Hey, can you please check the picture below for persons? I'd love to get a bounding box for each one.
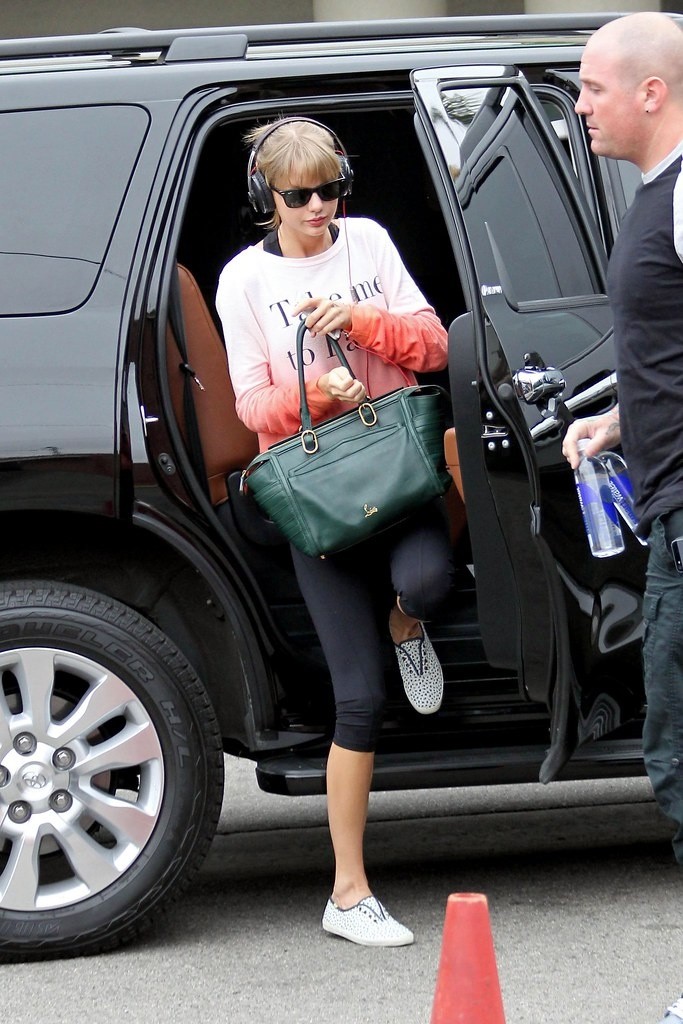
[562,14,683,859]
[205,114,472,945]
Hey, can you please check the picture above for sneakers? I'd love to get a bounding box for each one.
[391,619,444,714]
[322,897,413,947]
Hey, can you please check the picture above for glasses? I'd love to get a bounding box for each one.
[267,171,348,207]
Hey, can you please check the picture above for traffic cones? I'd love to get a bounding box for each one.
[429,891,508,1023]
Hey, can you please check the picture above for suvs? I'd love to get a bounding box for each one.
[0,8,682,964]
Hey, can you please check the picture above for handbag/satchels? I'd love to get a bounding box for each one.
[238,317,452,560]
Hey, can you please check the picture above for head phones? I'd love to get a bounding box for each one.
[247,116,355,214]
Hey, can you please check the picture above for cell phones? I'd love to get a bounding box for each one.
[293,294,342,341]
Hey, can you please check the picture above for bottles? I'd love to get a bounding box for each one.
[573,437,626,558]
[595,445,651,548]
